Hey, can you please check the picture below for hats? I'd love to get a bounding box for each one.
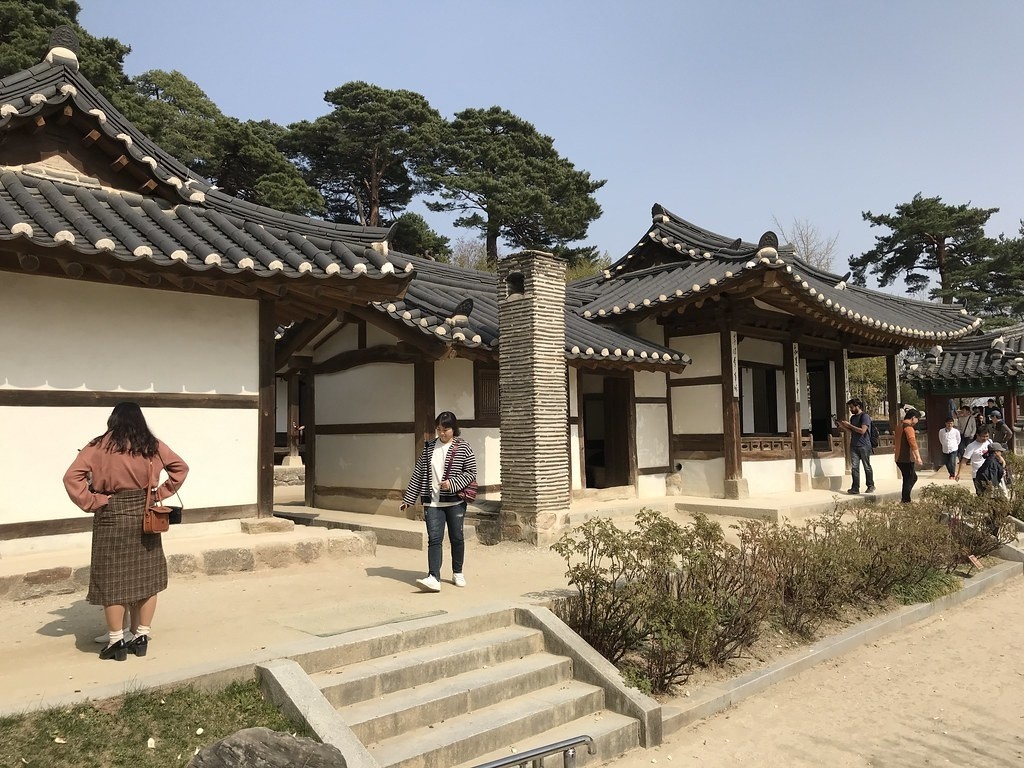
[988,443,1004,452]
[988,410,1001,417]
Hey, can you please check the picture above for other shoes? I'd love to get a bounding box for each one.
[865,486,875,493]
[848,488,859,494]
[966,460,972,465]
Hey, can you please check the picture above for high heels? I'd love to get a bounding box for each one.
[126,635,148,656]
[99,639,128,660]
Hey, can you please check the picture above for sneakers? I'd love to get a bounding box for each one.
[95,627,129,643]
[123,630,134,642]
[452,572,465,586]
[416,574,441,593]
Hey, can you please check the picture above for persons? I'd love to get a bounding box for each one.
[834,398,876,495]
[62,402,190,661]
[991,410,1013,448]
[399,411,477,592]
[895,409,922,503]
[938,417,962,479]
[977,443,1009,499]
[956,399,1003,465]
[963,424,994,485]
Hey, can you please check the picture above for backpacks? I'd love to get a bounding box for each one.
[851,413,879,447]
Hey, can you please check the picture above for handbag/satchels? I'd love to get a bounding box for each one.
[961,433,965,442]
[165,506,182,524]
[142,506,173,533]
[445,438,478,503]
